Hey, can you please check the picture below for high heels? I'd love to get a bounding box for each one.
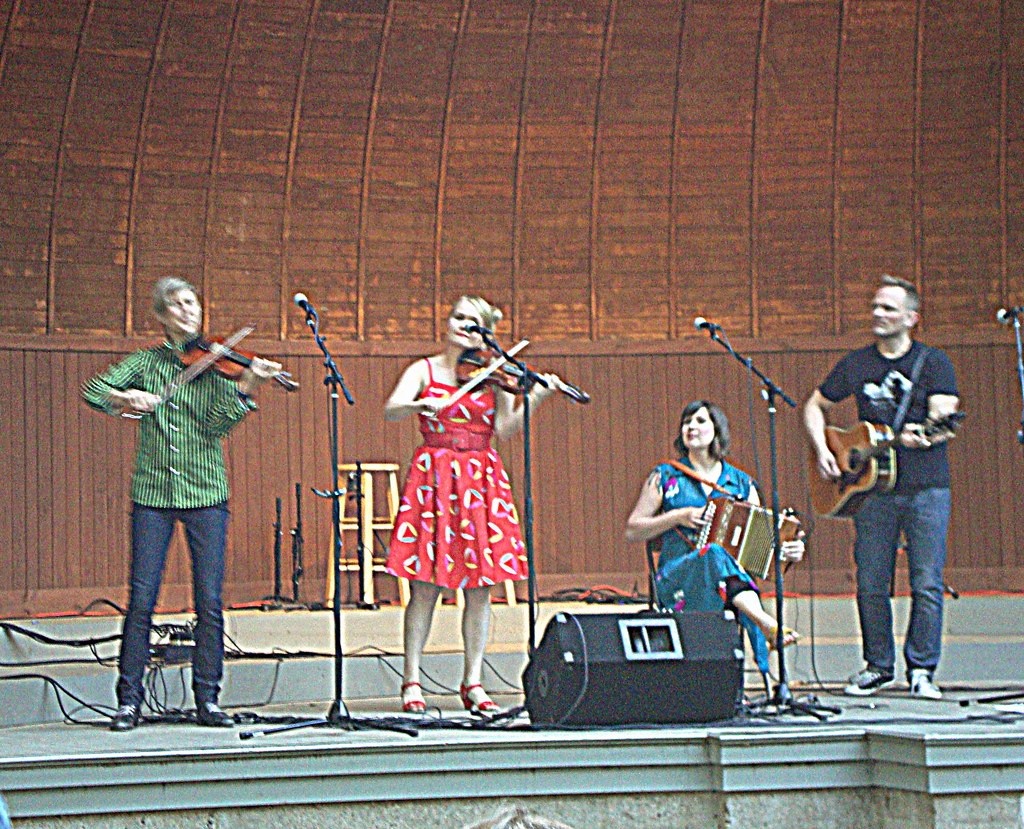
[400,680,427,714]
[459,681,501,712]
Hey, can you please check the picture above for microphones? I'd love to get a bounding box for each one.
[294,292,317,317]
[996,307,1023,324]
[465,321,493,335]
[693,317,721,332]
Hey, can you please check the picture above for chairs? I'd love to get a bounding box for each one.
[637,528,773,700]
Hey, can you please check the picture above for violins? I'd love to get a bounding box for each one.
[453,344,591,405]
[175,332,302,393]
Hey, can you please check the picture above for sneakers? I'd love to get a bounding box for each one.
[195,702,235,727]
[110,704,142,731]
[844,668,895,695]
[906,668,942,700]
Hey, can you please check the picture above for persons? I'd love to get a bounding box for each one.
[625,399,806,675]
[803,276,959,701]
[382,295,560,713]
[77,277,283,732]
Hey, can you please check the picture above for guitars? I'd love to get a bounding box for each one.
[805,407,973,520]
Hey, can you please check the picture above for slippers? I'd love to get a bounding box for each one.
[771,628,802,650]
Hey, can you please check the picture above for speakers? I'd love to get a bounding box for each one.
[522,610,745,729]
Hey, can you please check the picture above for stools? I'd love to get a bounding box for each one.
[325,460,410,606]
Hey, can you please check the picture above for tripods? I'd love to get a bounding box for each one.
[705,331,841,722]
[238,316,418,741]
